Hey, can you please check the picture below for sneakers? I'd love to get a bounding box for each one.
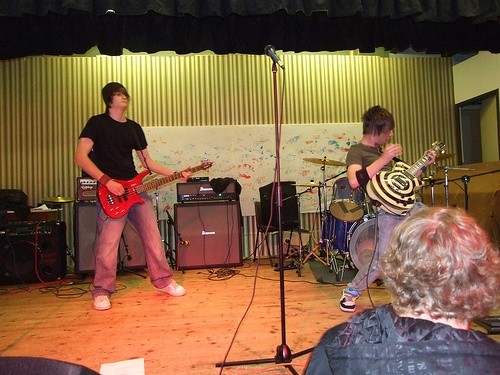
[153,278,186,297]
[339,288,360,312]
[92,295,112,311]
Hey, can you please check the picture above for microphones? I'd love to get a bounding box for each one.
[263,45,285,70]
[179,239,190,246]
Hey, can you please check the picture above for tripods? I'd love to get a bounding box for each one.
[214,62,315,375]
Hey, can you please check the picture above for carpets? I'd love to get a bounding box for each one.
[307,256,383,284]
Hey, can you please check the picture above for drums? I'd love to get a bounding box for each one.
[318,210,354,254]
[328,177,365,223]
[346,214,379,270]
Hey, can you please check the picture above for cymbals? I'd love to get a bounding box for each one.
[291,183,333,188]
[41,196,75,204]
[428,152,457,163]
[302,158,347,166]
[420,177,446,183]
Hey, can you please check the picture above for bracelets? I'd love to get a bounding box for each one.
[356,167,370,186]
[99,174,111,186]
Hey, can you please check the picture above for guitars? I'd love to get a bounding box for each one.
[366,141,446,217]
[97,159,213,219]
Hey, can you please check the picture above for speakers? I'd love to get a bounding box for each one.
[74,202,147,273]
[0,232,66,285]
[173,201,243,268]
[260,181,299,228]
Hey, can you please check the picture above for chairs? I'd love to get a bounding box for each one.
[252,201,278,268]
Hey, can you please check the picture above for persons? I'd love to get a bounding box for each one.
[340,104,437,311]
[75,82,190,310]
[304,206,500,375]
